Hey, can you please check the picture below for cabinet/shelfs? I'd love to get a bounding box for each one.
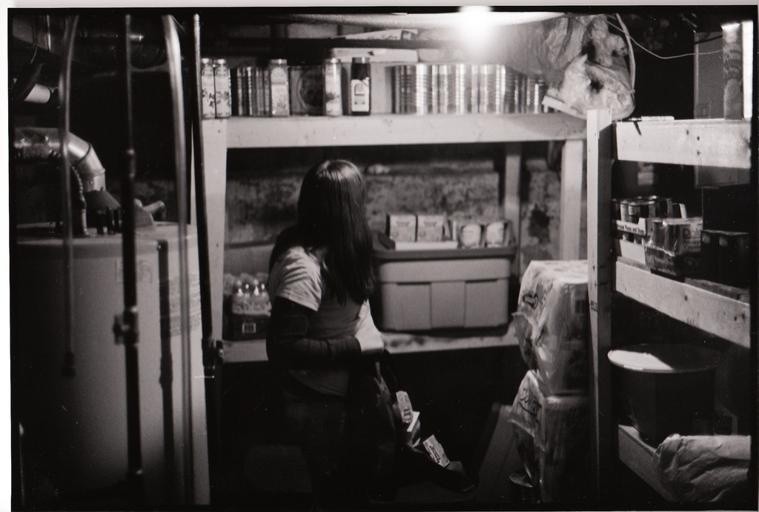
[197,109,584,512]
[587,111,759,511]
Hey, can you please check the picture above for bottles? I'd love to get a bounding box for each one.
[269,59,290,116]
[350,56,371,116]
[213,58,232,118]
[321,58,343,116]
[201,58,216,118]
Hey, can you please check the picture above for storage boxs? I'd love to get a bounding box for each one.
[373,228,511,332]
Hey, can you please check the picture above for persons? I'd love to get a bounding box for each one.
[265,158,385,399]
